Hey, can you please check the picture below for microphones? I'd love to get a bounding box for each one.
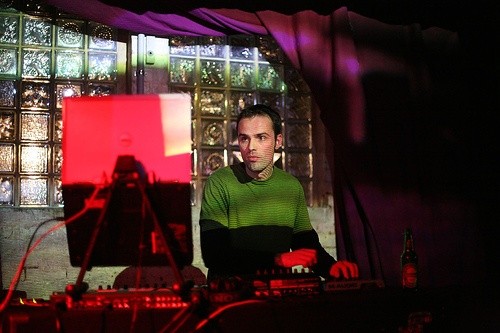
[401,230,419,288]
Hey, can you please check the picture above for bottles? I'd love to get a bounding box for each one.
[400,228,417,292]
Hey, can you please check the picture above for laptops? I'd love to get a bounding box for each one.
[63,93,195,268]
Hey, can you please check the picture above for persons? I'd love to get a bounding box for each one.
[200,105,359,279]
